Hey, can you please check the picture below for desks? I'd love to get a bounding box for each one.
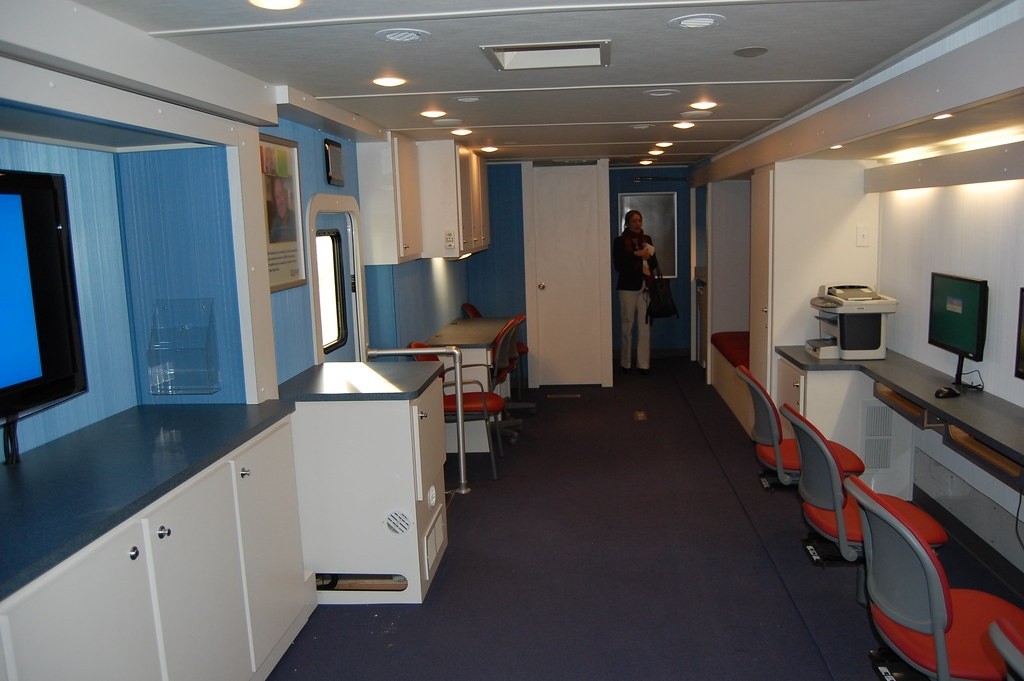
[425,315,514,393]
[775,346,1024,495]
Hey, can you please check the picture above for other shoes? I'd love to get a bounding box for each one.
[638,368,652,375]
[621,367,631,374]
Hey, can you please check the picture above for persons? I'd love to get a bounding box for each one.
[613,210,659,375]
[268,176,297,241]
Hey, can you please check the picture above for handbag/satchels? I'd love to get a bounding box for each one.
[642,253,679,324]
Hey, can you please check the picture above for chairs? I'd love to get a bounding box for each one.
[408,302,528,482]
[844,477,1024,681]
[779,405,948,606]
[735,362,865,485]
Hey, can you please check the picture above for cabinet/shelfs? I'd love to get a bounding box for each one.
[0,379,448,681]
[357,140,491,266]
[776,358,914,501]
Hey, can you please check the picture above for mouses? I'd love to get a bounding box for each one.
[934,386,962,398]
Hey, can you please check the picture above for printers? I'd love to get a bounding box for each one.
[805,283,899,360]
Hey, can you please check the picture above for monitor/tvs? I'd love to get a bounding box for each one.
[927,272,989,385]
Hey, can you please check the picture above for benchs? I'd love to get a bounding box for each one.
[710,332,755,440]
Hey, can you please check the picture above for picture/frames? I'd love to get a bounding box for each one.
[259,133,307,294]
[618,191,679,282]
